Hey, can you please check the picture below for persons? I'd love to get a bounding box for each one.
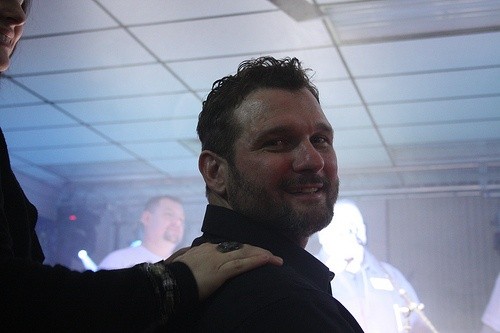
[189,54,363,333]
[92,194,185,270]
[315,201,421,333]
[0,0,287,333]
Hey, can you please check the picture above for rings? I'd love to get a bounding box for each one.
[215,239,245,253]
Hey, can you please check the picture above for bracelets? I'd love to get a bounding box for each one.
[142,258,179,310]
